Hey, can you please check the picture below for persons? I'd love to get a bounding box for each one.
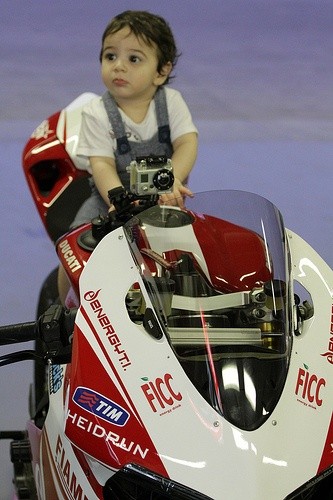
[56,10,199,310]
